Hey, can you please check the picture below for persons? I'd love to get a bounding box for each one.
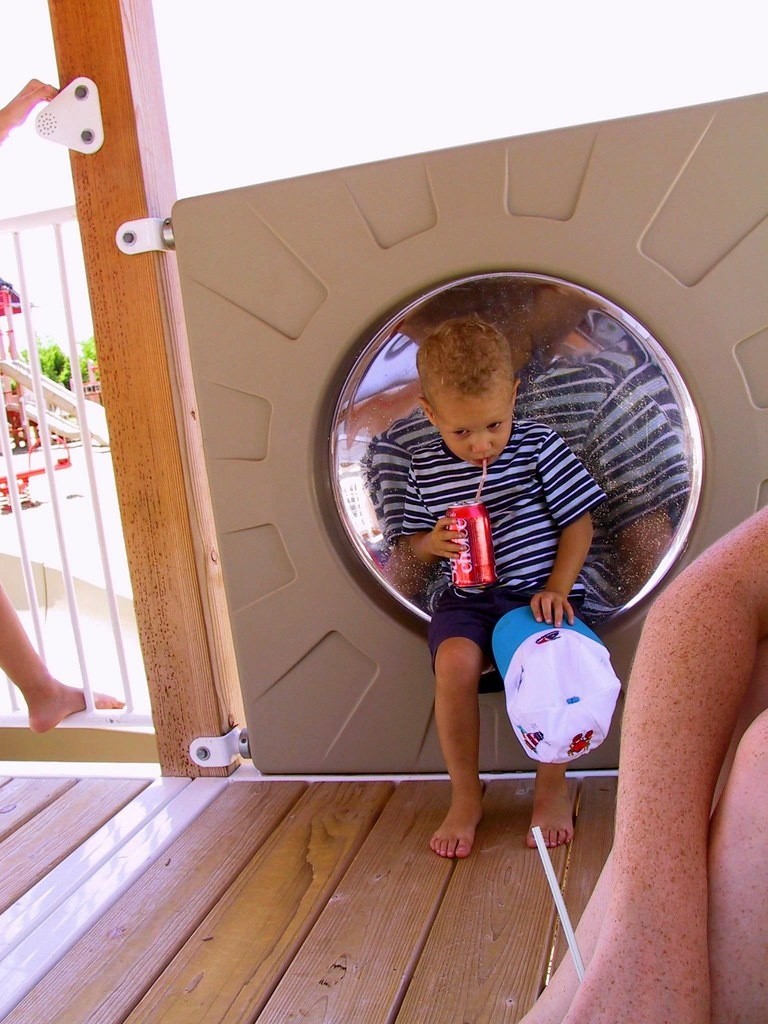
[0,579,124,733]
[517,498,768,1024]
[361,282,695,601]
[360,320,619,860]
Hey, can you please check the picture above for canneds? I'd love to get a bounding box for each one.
[445,500,497,589]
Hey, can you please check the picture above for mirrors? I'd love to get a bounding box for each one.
[316,267,709,653]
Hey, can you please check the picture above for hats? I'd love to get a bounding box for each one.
[492,605,620,764]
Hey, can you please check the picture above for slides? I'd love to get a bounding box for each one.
[1,359,112,447]
[6,400,82,440]
